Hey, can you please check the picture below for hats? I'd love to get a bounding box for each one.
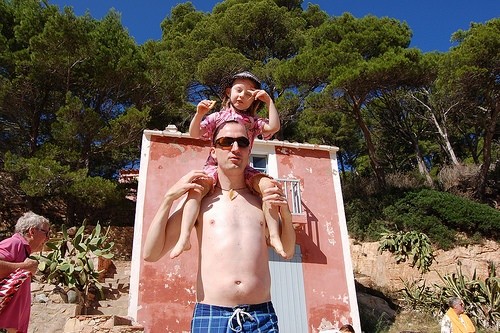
[233,71,261,84]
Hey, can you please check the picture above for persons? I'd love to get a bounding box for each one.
[440,297,476,333]
[0,211,51,333]
[170,70,287,258]
[319,324,357,333]
[144,119,296,333]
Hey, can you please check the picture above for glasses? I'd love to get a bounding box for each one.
[212,136,250,148]
[457,302,463,307]
[38,228,49,237]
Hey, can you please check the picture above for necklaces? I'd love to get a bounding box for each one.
[216,185,248,200]
